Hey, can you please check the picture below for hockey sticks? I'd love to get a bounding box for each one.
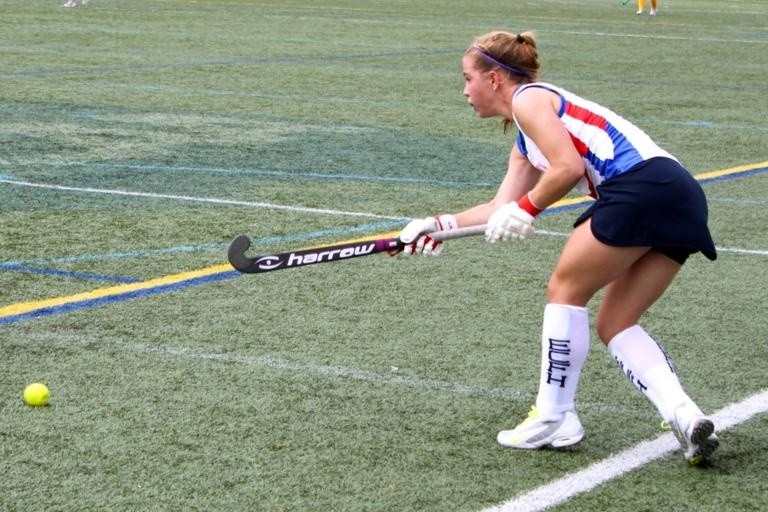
[227,222,486,272]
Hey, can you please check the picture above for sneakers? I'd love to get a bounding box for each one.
[666,402,720,466]
[497,405,587,451]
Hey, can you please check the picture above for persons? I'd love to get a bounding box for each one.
[635,0,657,17]
[398,31,722,466]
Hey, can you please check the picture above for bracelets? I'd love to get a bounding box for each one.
[518,192,542,218]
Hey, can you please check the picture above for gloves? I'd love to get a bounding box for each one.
[485,192,544,244]
[390,214,457,257]
